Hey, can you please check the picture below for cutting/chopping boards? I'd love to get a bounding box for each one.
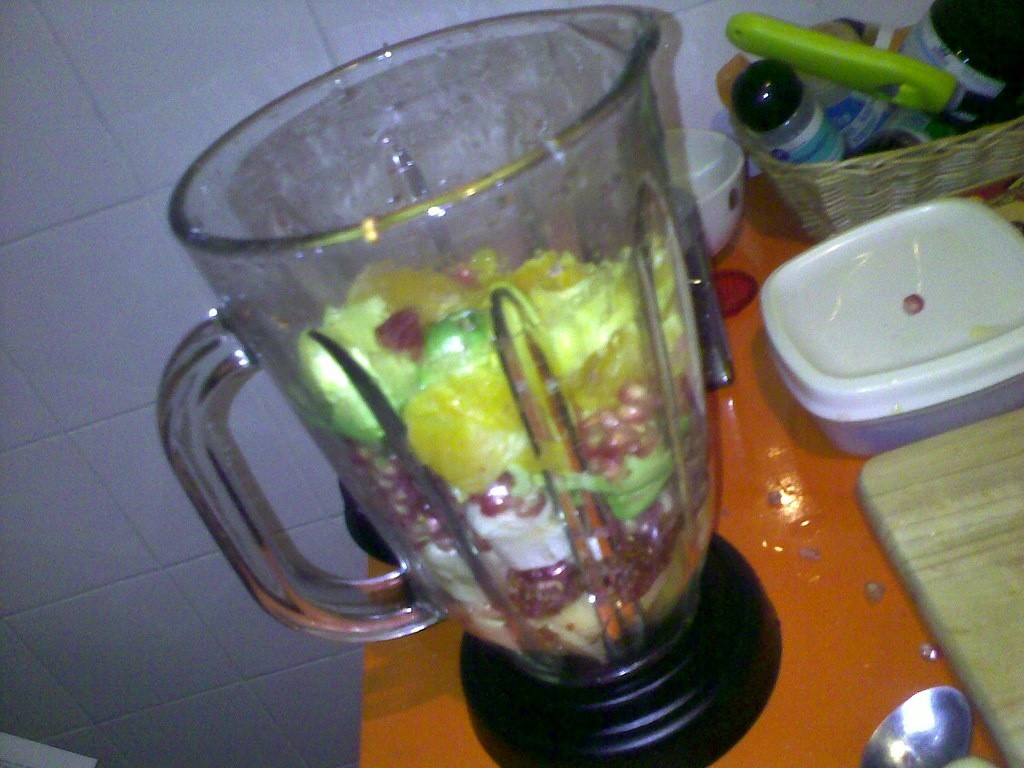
[856,408,1024,768]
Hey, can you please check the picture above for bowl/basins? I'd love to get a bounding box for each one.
[668,130,747,258]
[760,195,1024,458]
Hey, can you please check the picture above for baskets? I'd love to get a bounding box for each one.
[729,115,1024,242]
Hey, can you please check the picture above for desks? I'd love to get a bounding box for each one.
[354,170,1024,768]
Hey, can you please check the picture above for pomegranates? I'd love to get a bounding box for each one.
[347,363,710,622]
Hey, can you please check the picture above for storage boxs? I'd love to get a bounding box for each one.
[758,195,1024,460]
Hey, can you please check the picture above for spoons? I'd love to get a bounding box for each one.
[861,687,973,768]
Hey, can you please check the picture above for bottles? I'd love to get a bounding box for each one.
[733,57,846,166]
[897,0,1024,129]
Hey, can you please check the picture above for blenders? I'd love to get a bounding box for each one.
[157,4,783,768]
